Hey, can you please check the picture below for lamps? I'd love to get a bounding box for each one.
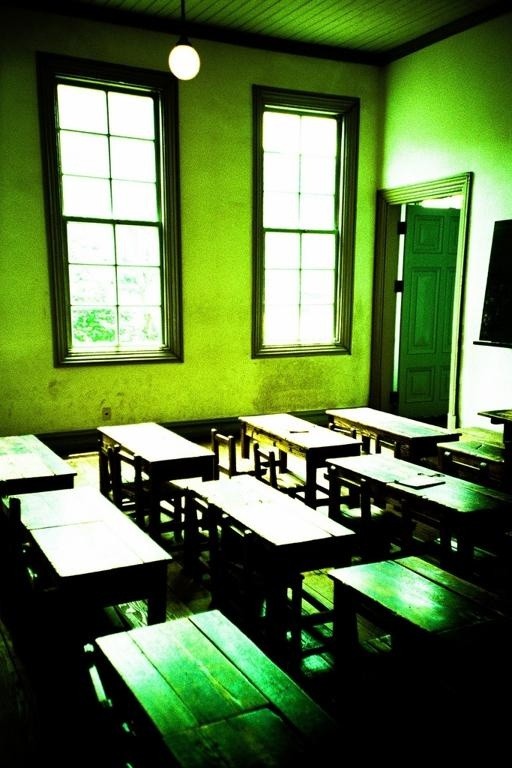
[167,0,201,81]
